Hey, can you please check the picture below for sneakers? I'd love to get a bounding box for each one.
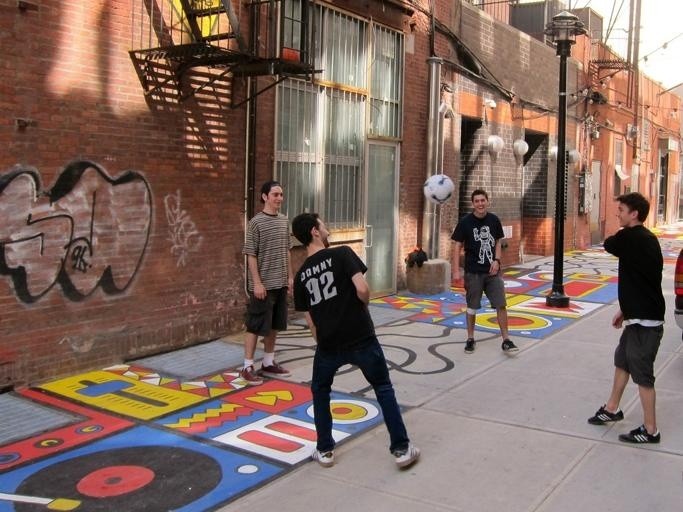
[619,424,660,444]
[262,359,290,376]
[502,339,519,352]
[588,403,624,423]
[395,444,420,467]
[464,338,474,353]
[312,449,334,467]
[241,365,262,386]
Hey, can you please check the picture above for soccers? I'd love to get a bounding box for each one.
[424,174,454,204]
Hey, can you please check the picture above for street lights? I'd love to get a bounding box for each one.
[544,8,586,306]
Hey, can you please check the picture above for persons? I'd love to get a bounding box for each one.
[289,211,423,472]
[586,191,665,446]
[238,177,294,384]
[447,187,521,357]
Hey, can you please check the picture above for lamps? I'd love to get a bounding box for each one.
[568,150,579,163]
[551,145,559,162]
[488,135,503,154]
[513,138,529,156]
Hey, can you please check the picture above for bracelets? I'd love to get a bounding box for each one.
[493,258,500,271]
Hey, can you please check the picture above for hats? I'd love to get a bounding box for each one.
[260,181,280,203]
